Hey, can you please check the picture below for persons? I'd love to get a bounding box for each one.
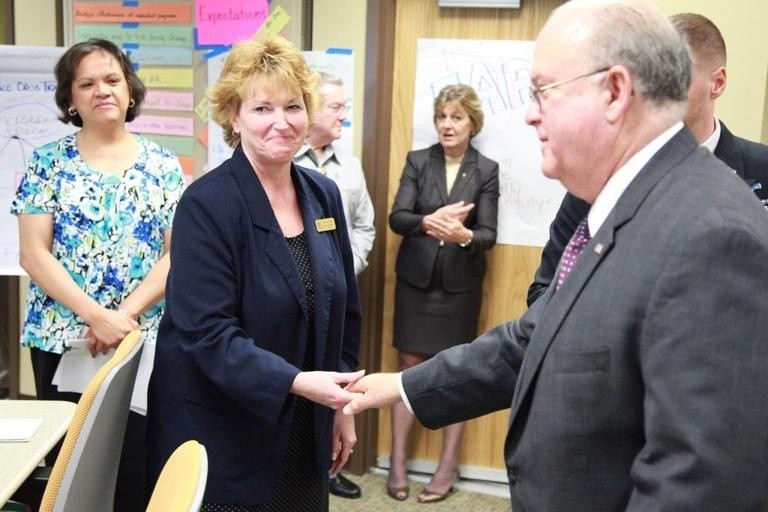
[140,33,368,509]
[291,70,378,497]
[333,2,766,509]
[385,84,498,504]
[10,40,190,511]
[525,17,766,308]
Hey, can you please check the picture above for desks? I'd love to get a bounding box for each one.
[1,398,79,511]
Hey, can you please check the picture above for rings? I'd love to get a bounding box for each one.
[349,449,353,453]
[439,233,444,239]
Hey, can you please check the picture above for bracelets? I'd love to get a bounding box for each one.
[460,230,473,247]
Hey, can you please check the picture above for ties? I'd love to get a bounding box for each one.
[555,217,591,296]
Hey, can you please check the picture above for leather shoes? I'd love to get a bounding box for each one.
[417,464,460,503]
[328,468,361,499]
[387,455,410,501]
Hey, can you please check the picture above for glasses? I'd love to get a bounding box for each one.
[528,66,611,114]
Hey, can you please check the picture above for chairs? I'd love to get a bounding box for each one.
[0,330,146,512]
[146,439,209,512]
